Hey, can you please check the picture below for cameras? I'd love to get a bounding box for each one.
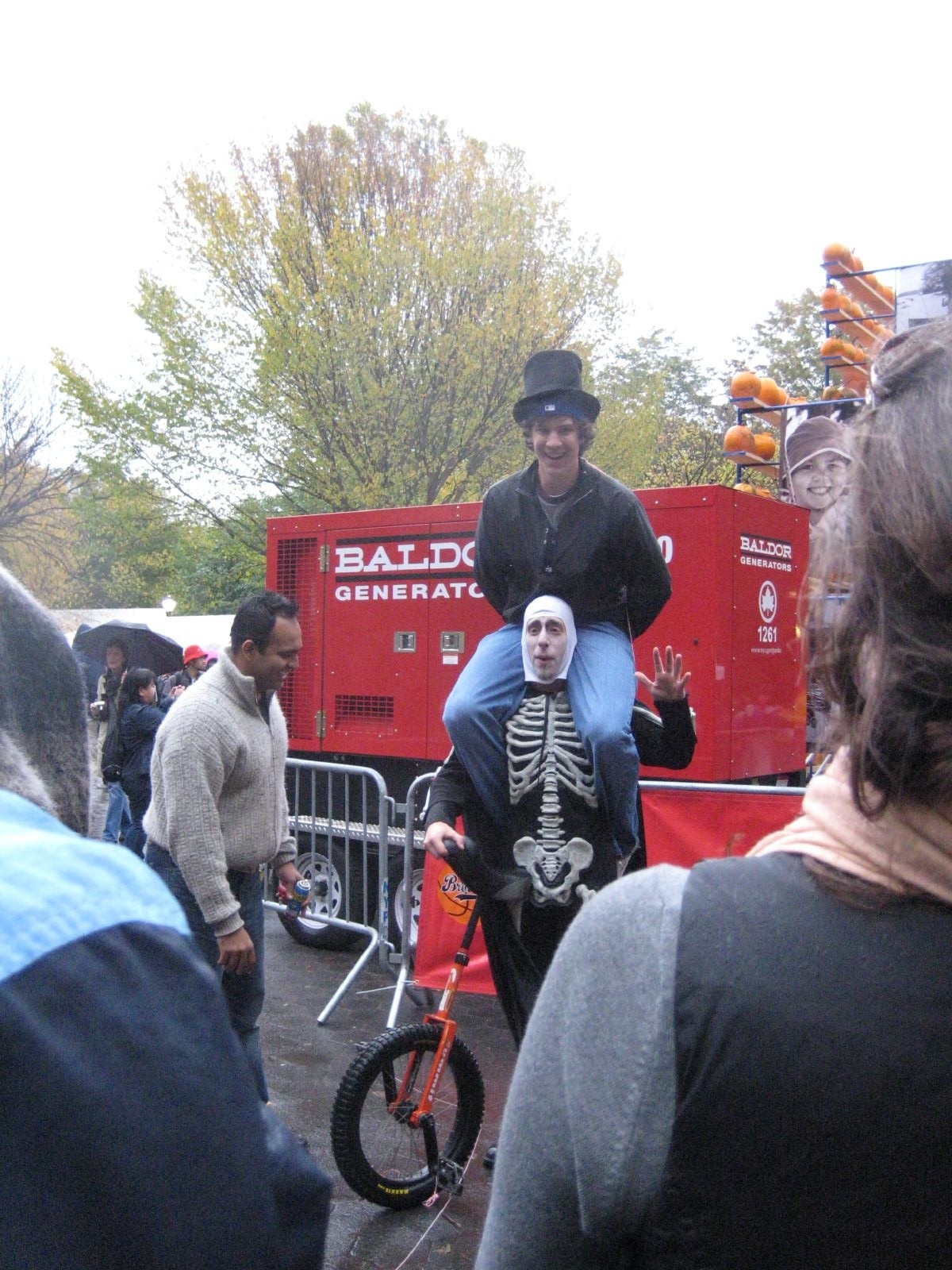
[94,701,106,710]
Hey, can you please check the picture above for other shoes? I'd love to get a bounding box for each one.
[483,1144,496,1166]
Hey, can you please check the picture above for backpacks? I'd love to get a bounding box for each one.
[101,709,124,782]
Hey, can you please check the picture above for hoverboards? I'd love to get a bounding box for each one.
[331,831,531,1213]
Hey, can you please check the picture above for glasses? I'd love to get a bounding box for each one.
[805,596,849,629]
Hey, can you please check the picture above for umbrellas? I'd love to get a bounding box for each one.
[78,618,185,680]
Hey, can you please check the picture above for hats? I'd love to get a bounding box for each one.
[782,416,855,488]
[527,396,585,420]
[184,645,208,665]
[513,351,601,426]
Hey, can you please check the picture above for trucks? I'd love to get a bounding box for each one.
[258,476,818,966]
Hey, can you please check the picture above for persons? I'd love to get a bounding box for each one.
[442,351,672,885]
[73,613,223,860]
[781,407,851,573]
[424,596,699,1170]
[142,593,312,1150]
[0,561,334,1270]
[474,318,952,1270]
